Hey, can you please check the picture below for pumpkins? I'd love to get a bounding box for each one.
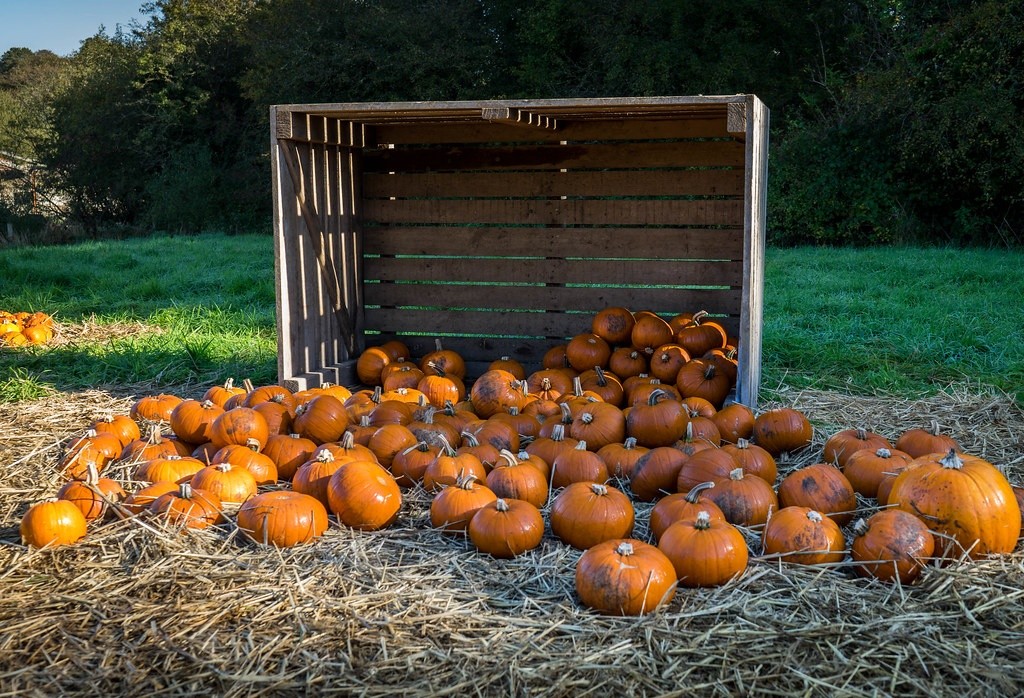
[0,310,54,347]
[19,307,1024,615]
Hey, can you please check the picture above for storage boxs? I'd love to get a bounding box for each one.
[268,95,771,421]
